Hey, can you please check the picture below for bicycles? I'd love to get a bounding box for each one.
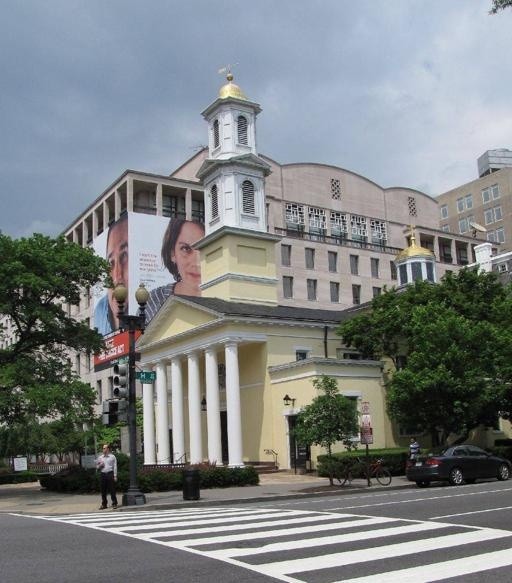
[351,458,393,486]
[328,456,370,486]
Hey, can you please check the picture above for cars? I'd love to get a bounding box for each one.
[405,444,510,486]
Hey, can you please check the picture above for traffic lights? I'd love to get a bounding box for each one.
[113,363,130,401]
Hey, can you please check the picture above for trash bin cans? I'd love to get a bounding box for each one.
[182,468,200,500]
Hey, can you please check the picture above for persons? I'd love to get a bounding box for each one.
[408,436,420,460]
[136,218,205,327]
[93,215,129,337]
[95,444,118,509]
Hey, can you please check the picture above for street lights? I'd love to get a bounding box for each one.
[114,281,150,504]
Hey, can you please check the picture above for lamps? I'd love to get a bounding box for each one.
[283,394,295,407]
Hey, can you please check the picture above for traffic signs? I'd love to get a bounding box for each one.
[359,400,374,444]
[134,370,157,381]
[117,354,131,363]
